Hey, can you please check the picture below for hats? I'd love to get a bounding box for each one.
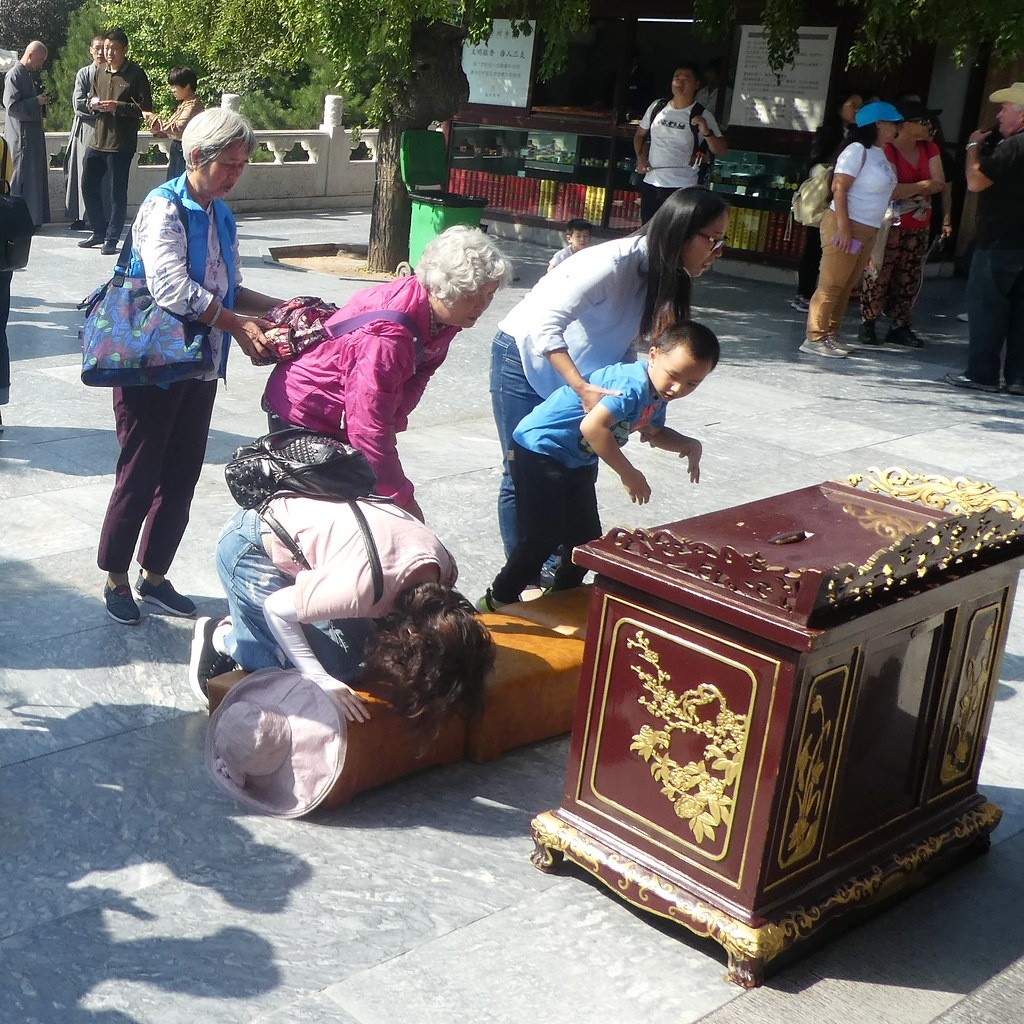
[204,667,348,819]
[896,100,943,123]
[989,83,1024,105]
[855,102,904,128]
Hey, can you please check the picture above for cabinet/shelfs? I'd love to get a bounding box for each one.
[528,465,1024,990]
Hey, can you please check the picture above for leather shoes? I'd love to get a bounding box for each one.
[78,231,104,248]
[101,239,117,255]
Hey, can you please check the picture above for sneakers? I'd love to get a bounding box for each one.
[799,337,849,359]
[189,616,246,709]
[102,580,141,625]
[133,569,197,616]
[827,335,855,353]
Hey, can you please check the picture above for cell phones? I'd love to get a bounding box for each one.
[45,89,56,96]
[688,155,703,166]
[937,232,952,252]
[830,233,864,255]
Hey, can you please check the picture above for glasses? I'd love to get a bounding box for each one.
[696,229,729,253]
[900,119,932,125]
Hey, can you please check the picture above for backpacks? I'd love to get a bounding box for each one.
[0,135,36,272]
[224,427,452,605]
[792,146,866,228]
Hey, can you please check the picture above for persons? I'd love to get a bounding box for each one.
[490,186,730,602]
[3,41,51,226]
[799,102,904,359]
[0,136,14,431]
[142,67,206,182]
[547,218,592,271]
[261,224,514,525]
[188,491,495,722]
[634,66,728,226]
[63,32,150,254]
[945,83,1024,394]
[698,58,740,147]
[791,89,863,313]
[476,319,720,612]
[858,95,955,346]
[97,108,286,623]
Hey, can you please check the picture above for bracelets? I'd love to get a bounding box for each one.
[966,142,980,150]
[942,223,952,226]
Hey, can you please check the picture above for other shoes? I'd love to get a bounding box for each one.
[1005,380,1024,394]
[475,587,525,614]
[887,324,924,348]
[542,582,587,595]
[944,373,1000,392]
[860,325,885,345]
[790,295,810,312]
[957,312,968,321]
[68,218,86,230]
[533,565,560,588]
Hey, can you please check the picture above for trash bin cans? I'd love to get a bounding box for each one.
[395,127,489,280]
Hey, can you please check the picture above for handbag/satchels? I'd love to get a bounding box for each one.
[250,296,340,366]
[75,188,216,387]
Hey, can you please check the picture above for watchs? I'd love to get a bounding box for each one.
[703,129,715,137]
